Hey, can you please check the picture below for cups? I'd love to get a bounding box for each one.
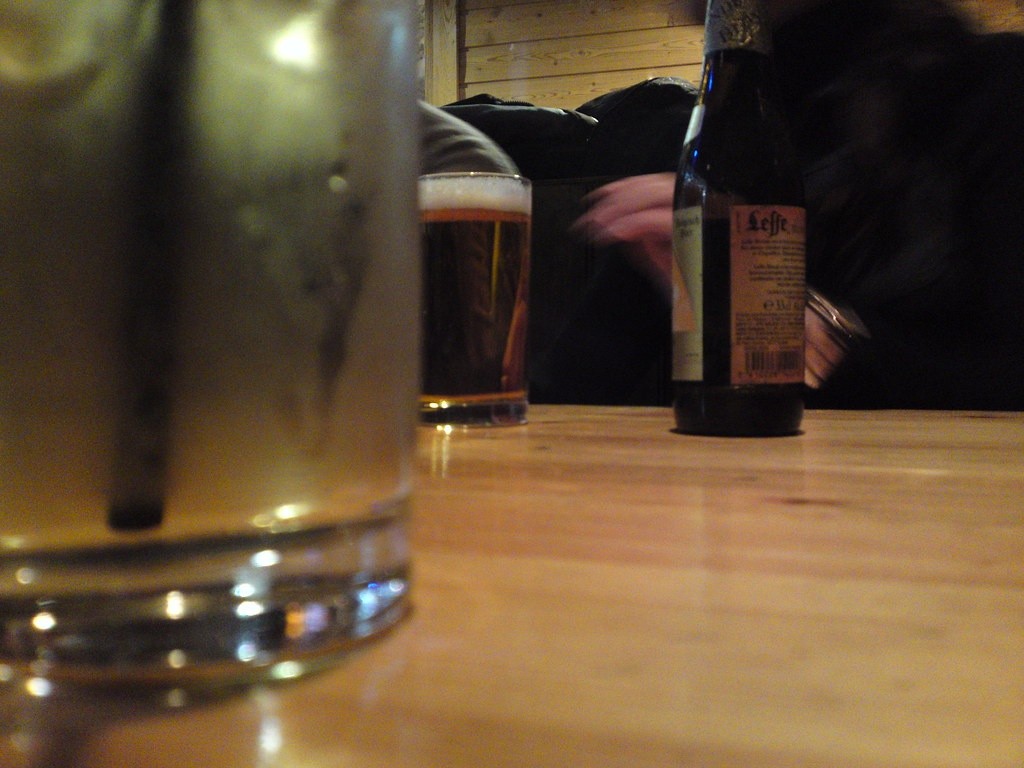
[416,175,532,423]
[0,1,421,683]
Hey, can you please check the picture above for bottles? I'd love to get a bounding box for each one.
[671,0,806,434]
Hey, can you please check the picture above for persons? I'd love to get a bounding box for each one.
[415,2,1024,412]
